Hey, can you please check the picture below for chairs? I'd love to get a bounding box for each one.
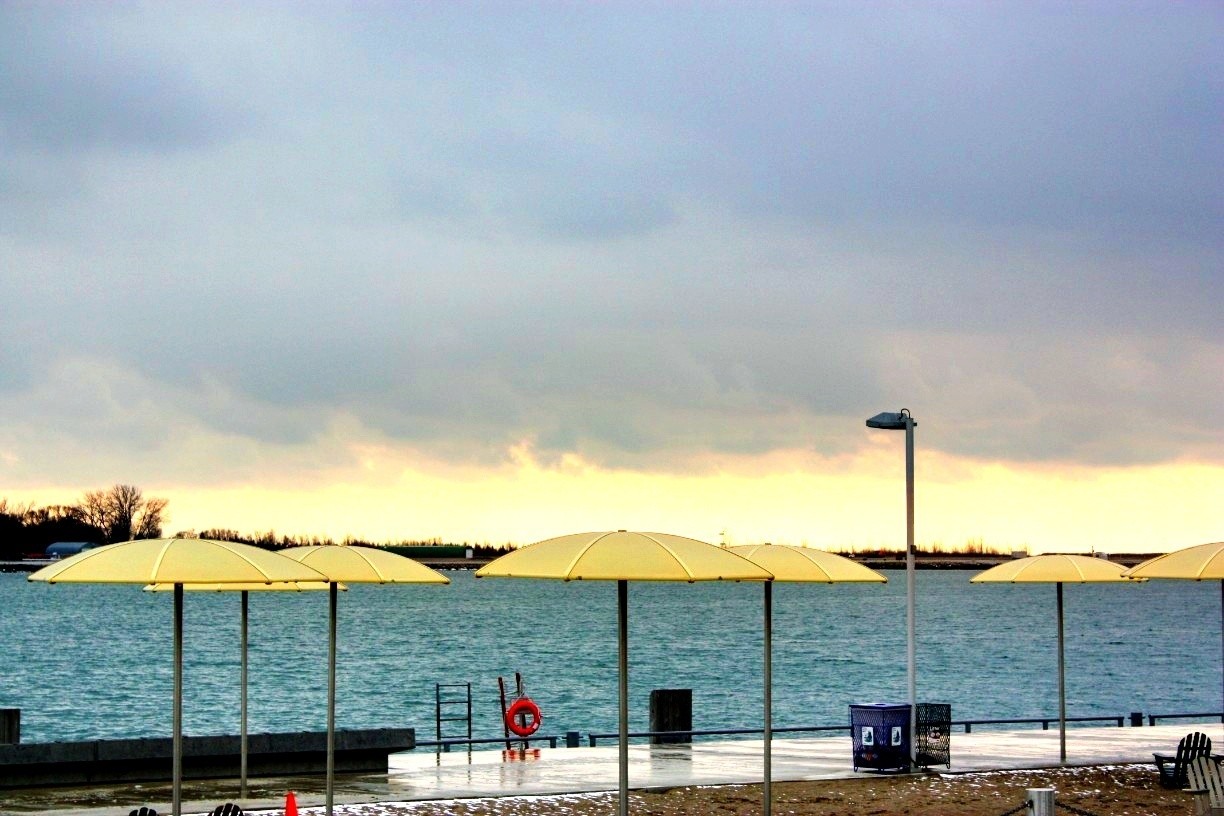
[1153,732,1224,785]
[1182,757,1224,816]
[436,682,472,751]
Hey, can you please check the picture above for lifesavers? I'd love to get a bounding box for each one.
[506,700,541,736]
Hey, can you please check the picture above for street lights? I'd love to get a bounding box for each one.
[863,408,924,775]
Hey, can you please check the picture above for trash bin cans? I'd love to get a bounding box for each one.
[849,703,912,774]
[648,689,692,743]
[916,703,953,769]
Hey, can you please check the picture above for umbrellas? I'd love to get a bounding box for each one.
[1122,541,1224,631]
[29,539,330,816]
[473,530,775,816]
[278,543,451,816]
[140,583,348,790]
[971,554,1149,760]
[726,543,888,816]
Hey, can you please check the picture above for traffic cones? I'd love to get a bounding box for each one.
[284,792,299,816]
